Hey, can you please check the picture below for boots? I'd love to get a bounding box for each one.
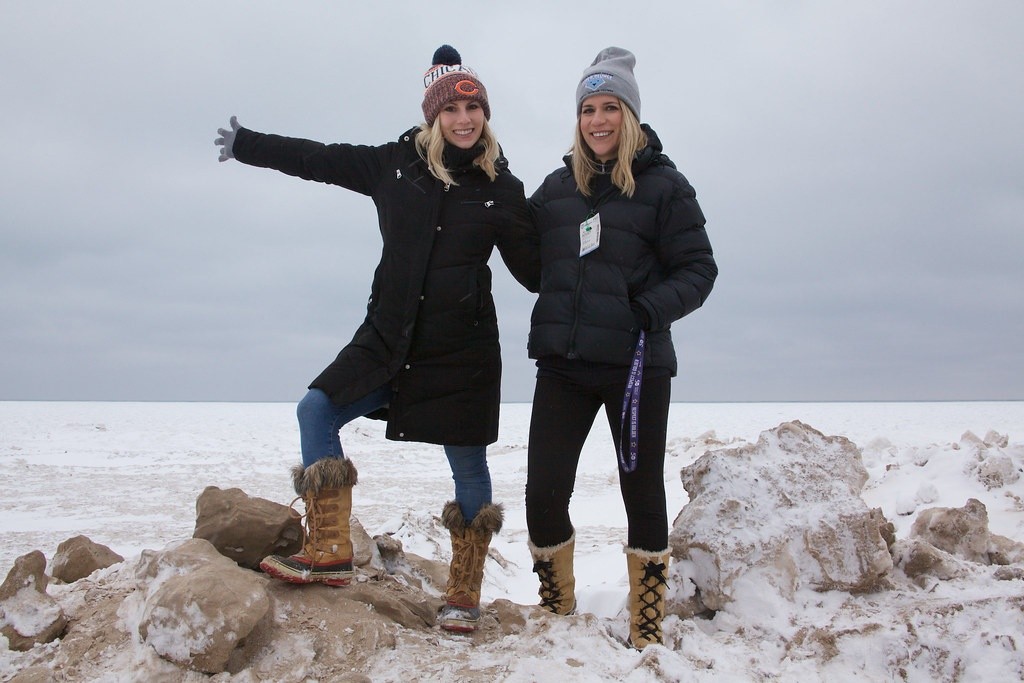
[439,502,505,632]
[261,456,358,583]
[529,525,577,617]
[626,546,672,654]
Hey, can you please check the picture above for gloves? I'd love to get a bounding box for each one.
[214,116,243,163]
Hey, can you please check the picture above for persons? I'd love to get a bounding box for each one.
[525,47,719,653]
[214,45,541,633]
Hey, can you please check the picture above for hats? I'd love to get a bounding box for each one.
[421,45,492,125]
[576,48,642,119]
